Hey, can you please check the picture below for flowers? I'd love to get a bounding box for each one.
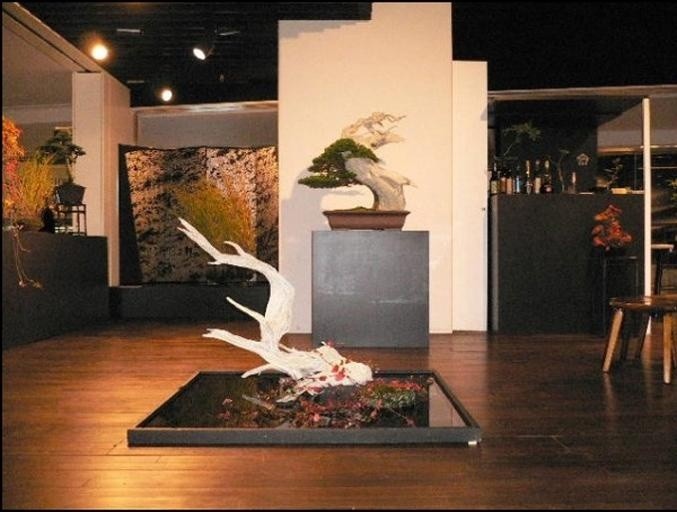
[590,205,632,252]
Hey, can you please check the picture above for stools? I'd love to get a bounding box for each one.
[601,294,677,384]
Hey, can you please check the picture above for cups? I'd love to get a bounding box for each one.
[489,159,580,193]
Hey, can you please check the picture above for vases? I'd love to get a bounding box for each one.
[604,246,627,257]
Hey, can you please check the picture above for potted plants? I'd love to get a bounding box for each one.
[34,132,86,203]
[297,112,420,229]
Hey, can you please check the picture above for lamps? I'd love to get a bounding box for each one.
[192,39,217,61]
